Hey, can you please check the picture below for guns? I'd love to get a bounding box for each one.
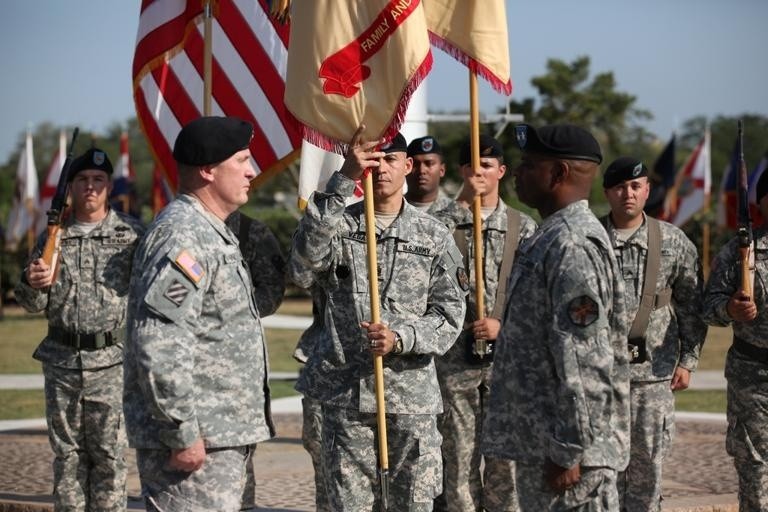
[737,117,754,303]
[41,126,79,294]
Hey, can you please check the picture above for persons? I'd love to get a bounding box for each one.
[122,115,275,512]
[434,136,539,512]
[702,170,768,512]
[287,123,471,512]
[404,135,453,214]
[489,123,632,512]
[226,211,286,318]
[598,157,708,512]
[13,147,148,512]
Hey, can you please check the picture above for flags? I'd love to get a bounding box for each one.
[297,79,428,211]
[421,0,513,178]
[133,0,302,198]
[643,119,768,232]
[285,0,433,179]
[5,131,172,254]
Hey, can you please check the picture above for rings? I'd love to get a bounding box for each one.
[371,339,376,347]
[566,485,573,489]
[480,331,483,336]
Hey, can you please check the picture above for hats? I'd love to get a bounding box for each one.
[458,134,503,166]
[602,156,649,188]
[173,116,252,169]
[66,147,113,181]
[378,129,441,157]
[514,123,603,162]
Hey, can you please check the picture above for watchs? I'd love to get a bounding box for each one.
[393,332,402,355]
